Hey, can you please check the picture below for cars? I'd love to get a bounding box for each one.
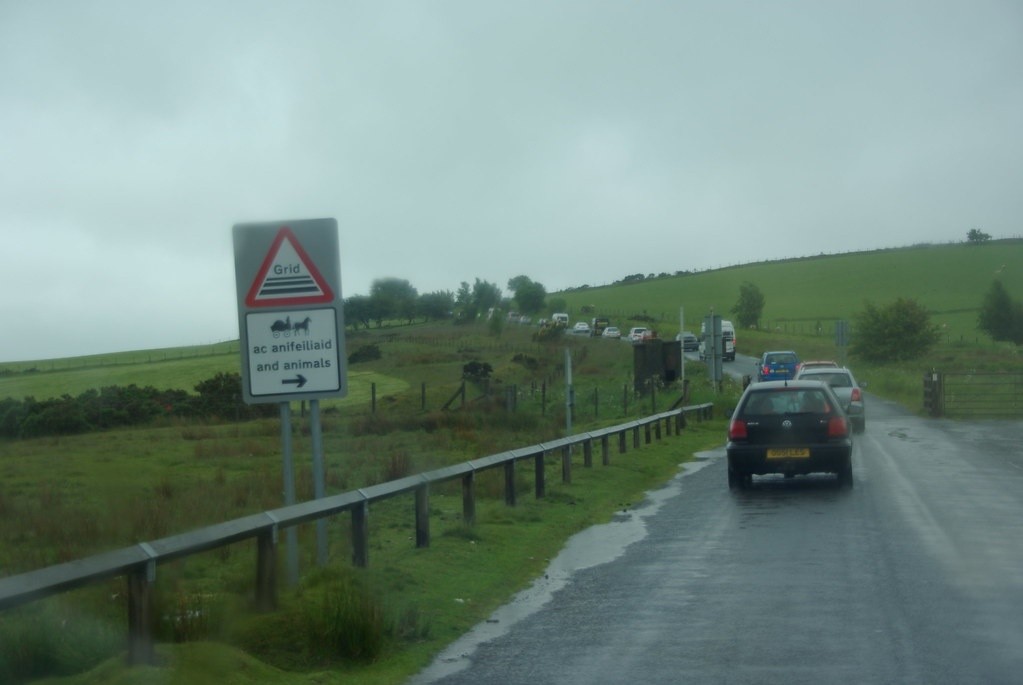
[487,306,551,328]
[675,332,699,351]
[601,327,621,339]
[627,327,649,342]
[724,378,855,491]
[573,322,590,334]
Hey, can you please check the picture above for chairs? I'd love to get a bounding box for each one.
[752,398,773,414]
[804,400,825,411]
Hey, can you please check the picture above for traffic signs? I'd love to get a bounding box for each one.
[231,217,349,405]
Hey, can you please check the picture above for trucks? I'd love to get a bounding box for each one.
[697,319,736,362]
[552,313,569,330]
[590,318,610,337]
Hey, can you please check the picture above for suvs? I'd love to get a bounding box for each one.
[793,366,866,433]
[795,359,839,375]
[754,351,800,382]
